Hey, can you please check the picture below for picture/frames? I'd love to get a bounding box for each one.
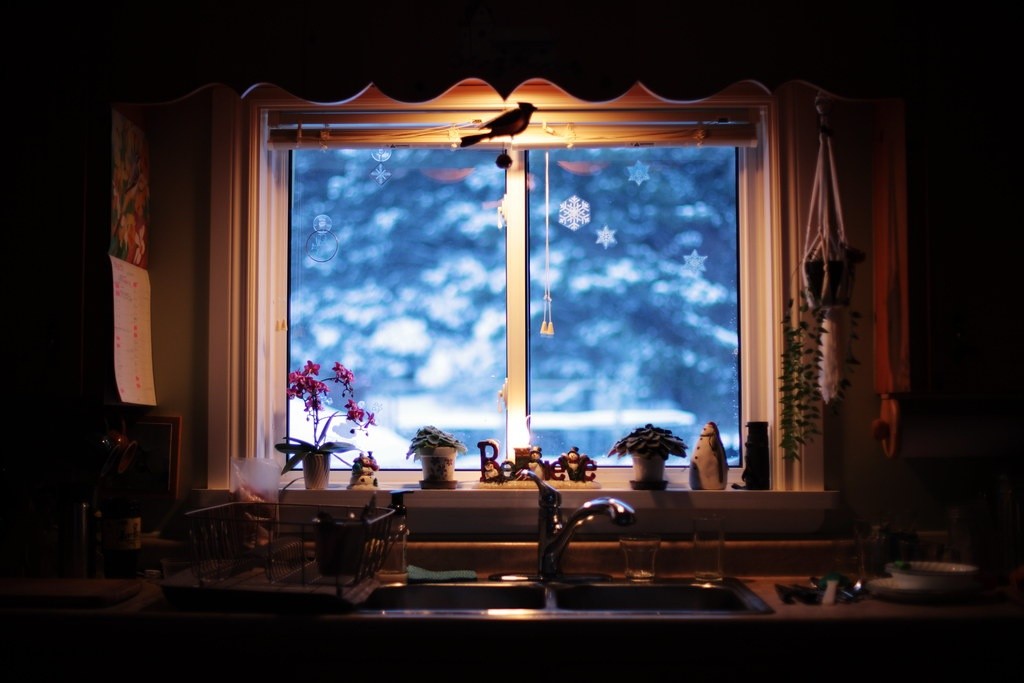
[108,415,183,506]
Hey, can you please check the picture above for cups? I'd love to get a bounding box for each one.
[619,535,660,580]
[691,514,724,581]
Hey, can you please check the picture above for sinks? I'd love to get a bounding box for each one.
[552,572,776,619]
[362,577,551,620]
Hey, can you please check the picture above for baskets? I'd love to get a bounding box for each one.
[184,502,396,588]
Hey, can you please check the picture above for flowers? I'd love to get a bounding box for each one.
[273,360,378,476]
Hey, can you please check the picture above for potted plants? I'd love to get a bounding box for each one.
[780,243,865,463]
[407,426,467,490]
[607,424,689,490]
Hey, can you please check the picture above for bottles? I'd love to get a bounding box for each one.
[689,422,728,491]
[101,475,143,576]
[745,422,771,489]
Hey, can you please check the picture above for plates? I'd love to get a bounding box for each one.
[867,578,985,602]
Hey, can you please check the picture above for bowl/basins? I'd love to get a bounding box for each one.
[885,561,977,592]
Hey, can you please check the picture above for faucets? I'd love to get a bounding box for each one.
[521,467,639,583]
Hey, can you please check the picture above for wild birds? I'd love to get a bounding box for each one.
[460,102,540,148]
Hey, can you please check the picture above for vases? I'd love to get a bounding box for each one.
[302,454,330,488]
